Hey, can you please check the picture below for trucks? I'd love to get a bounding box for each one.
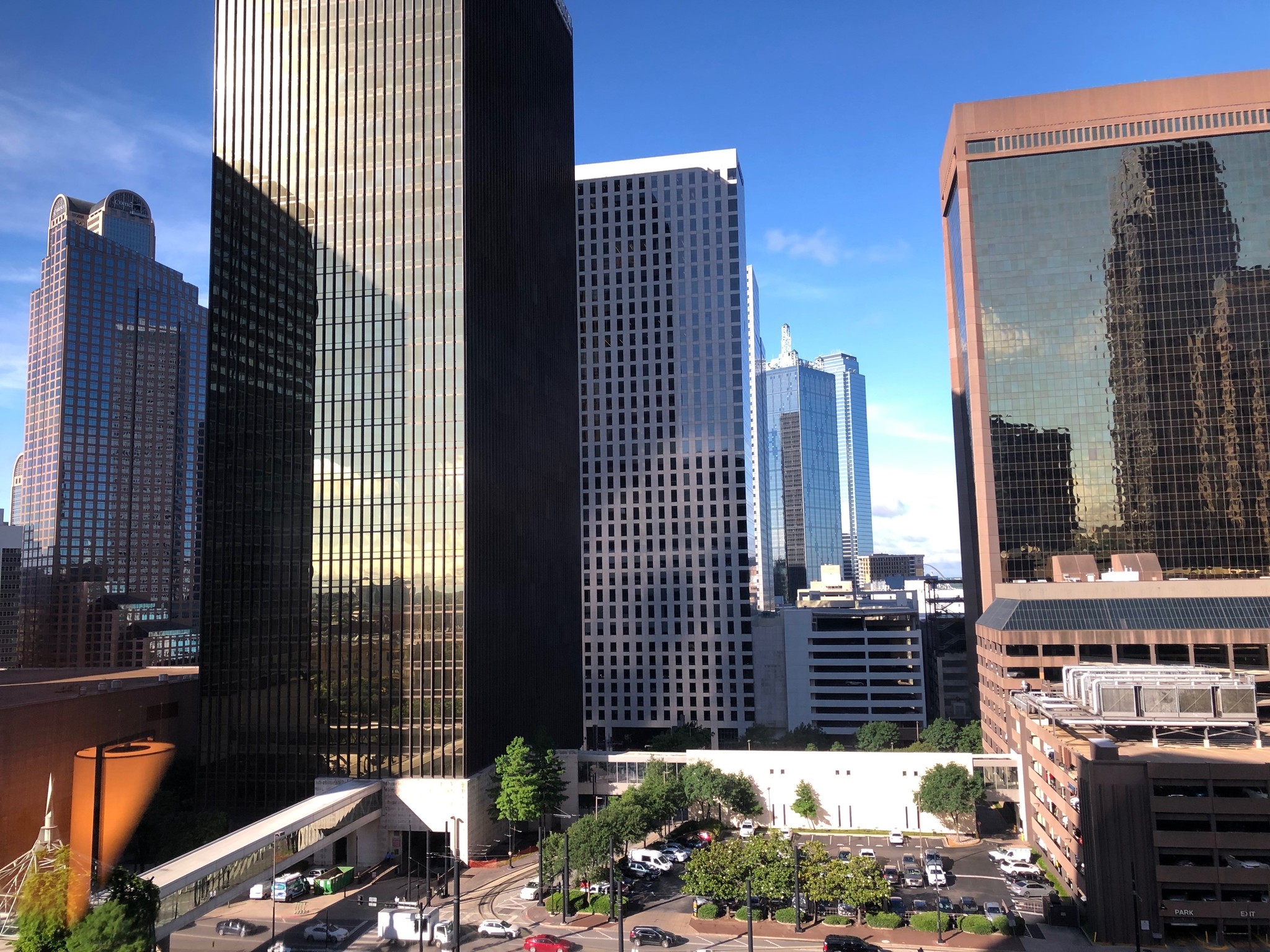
[378,906,454,949]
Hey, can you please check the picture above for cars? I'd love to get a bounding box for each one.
[580,826,1058,928]
[523,934,574,952]
[304,923,350,944]
[520,876,551,901]
[215,918,258,937]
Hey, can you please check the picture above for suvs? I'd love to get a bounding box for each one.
[630,925,677,948]
[821,934,885,952]
[739,820,755,837]
[478,918,522,939]
[249,880,273,900]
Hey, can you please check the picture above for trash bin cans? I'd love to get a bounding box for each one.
[440,889,444,899]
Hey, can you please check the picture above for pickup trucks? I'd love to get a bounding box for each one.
[305,868,329,887]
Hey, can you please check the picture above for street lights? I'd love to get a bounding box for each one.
[450,816,456,860]
[747,739,751,751]
[272,831,285,937]
[595,795,603,822]
[767,787,772,834]
[664,770,670,835]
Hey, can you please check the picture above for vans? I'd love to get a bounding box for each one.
[271,872,307,903]
